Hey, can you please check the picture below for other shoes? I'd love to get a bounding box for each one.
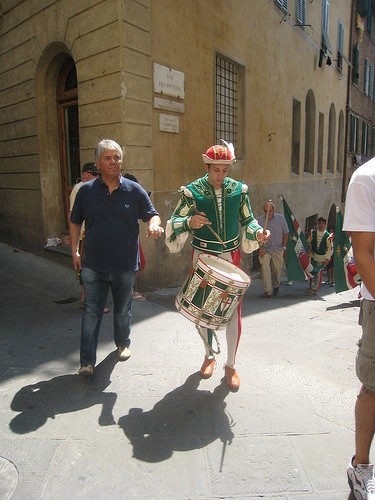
[326,281,335,286]
[305,288,318,295]
[131,289,145,302]
[273,287,279,296]
[79,301,110,313]
[260,292,271,298]
[287,280,292,286]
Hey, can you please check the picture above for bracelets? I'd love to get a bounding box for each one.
[282,246,287,250]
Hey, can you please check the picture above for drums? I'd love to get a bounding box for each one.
[174,252,252,331]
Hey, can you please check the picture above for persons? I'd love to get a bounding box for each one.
[165,138,271,390]
[70,139,164,377]
[341,155,375,500]
[68,161,144,313]
[255,202,289,298]
[306,217,334,296]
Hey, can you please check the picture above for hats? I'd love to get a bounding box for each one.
[82,161,97,171]
[201,138,236,165]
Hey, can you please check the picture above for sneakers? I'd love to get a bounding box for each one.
[346,454,375,500]
[117,346,131,360]
[78,364,94,377]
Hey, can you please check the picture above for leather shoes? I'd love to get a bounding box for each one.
[224,364,240,391]
[200,356,215,378]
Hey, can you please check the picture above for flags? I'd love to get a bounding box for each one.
[333,211,363,294]
[281,198,326,282]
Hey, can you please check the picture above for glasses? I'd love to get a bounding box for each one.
[87,171,102,176]
